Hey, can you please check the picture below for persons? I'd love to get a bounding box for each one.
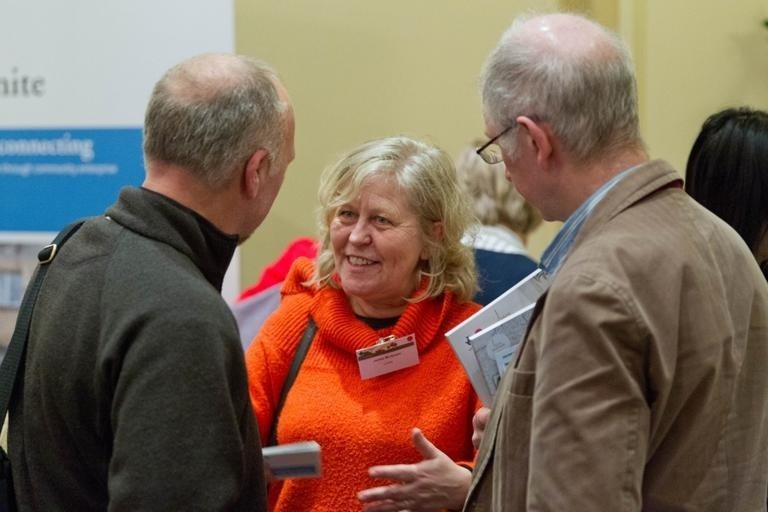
[472,14,768,512]
[0,53,296,512]
[245,137,483,512]
[459,148,541,306]
[685,107,768,283]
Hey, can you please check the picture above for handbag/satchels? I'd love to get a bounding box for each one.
[0,444,15,512]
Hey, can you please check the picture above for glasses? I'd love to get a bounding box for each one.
[476,125,513,165]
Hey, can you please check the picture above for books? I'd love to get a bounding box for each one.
[443,268,550,409]
